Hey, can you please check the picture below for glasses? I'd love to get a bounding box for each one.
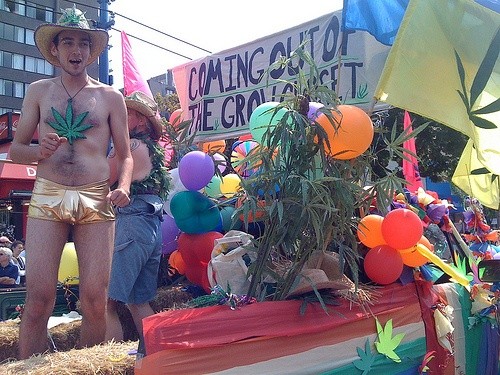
[0,253,6,255]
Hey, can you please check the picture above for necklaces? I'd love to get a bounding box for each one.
[60,76,90,102]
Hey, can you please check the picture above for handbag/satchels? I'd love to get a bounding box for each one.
[208,230,257,297]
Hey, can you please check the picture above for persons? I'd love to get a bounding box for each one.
[0,236,27,288]
[105,90,173,343]
[8,5,134,360]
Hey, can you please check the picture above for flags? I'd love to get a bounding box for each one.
[121,30,162,119]
[342,0,500,48]
[451,139,500,210]
[375,0,500,175]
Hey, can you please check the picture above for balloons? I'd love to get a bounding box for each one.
[200,139,226,154]
[363,244,403,285]
[400,235,434,267]
[57,242,79,284]
[381,209,423,250]
[162,153,265,294]
[169,109,185,128]
[358,215,388,248]
[307,102,326,123]
[417,243,469,286]
[310,105,374,160]
[249,101,295,147]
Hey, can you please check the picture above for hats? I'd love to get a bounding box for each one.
[284,268,351,300]
[290,250,346,289]
[34,7,109,69]
[0,236,12,244]
[122,90,162,142]
